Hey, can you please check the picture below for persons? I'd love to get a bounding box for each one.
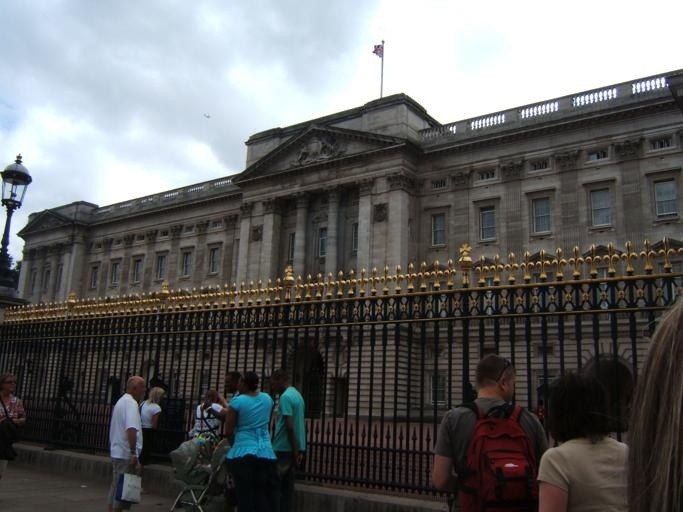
[139,386,166,493]
[0,373,27,501]
[629,299,683,511]
[107,376,147,512]
[536,370,629,512]
[203,371,243,505]
[188,390,230,505]
[270,367,305,511]
[431,353,549,512]
[225,371,274,511]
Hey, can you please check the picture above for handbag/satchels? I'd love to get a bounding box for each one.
[1,420,23,446]
[116,473,142,502]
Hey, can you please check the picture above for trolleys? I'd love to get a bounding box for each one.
[167,432,232,512]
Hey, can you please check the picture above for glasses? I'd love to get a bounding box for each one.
[497,361,511,381]
[5,380,16,383]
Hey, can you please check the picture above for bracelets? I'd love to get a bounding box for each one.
[129,447,136,453]
[205,406,212,411]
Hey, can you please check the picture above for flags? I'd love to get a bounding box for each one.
[372,44,382,57]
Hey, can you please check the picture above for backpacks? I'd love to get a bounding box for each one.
[456,402,540,511]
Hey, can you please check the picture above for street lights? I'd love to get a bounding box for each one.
[1,152,33,288]
